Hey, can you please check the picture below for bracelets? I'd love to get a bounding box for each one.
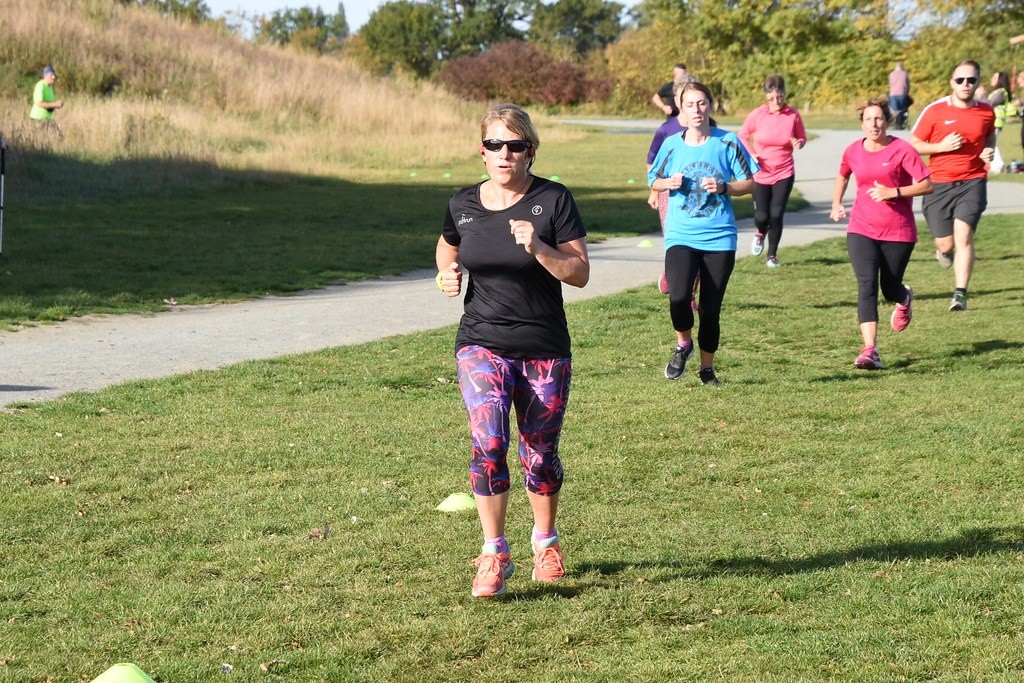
[895,187,901,199]
[718,182,727,194]
[436,271,444,292]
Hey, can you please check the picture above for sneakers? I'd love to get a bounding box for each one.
[664,339,695,380]
[766,256,781,269]
[752,234,765,256]
[890,284,913,333]
[949,290,967,310]
[855,347,881,368]
[696,368,720,386]
[471,540,515,597]
[937,250,954,269]
[531,534,565,582]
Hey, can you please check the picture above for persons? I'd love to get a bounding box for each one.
[647,81,760,386]
[647,74,721,311]
[29,66,65,143]
[652,63,688,120]
[908,60,998,312]
[888,61,911,130]
[829,101,934,369]
[973,71,1012,174]
[1014,71,1024,149]
[738,75,806,268]
[436,104,592,597]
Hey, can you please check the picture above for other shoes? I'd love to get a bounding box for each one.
[691,300,698,313]
[658,272,670,294]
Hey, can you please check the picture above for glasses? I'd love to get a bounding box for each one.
[955,77,977,85]
[483,138,530,152]
